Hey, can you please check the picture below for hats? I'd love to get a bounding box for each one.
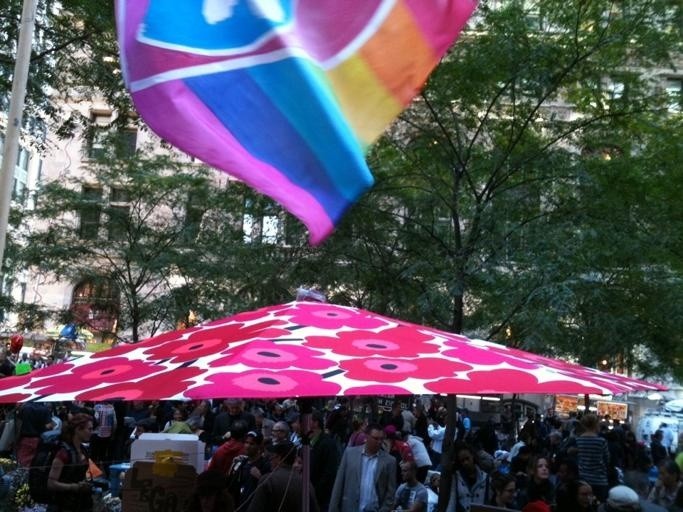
[606,485,639,512]
[266,441,296,459]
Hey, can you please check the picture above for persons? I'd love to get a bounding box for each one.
[1,395,682,512]
[0,342,76,378]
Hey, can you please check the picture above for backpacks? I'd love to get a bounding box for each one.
[28,438,91,505]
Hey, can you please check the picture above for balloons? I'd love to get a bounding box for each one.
[60,322,75,338]
[9,336,23,353]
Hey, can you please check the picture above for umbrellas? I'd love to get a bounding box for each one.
[0,282,667,512]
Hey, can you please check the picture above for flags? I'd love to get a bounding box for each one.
[111,2,477,245]
